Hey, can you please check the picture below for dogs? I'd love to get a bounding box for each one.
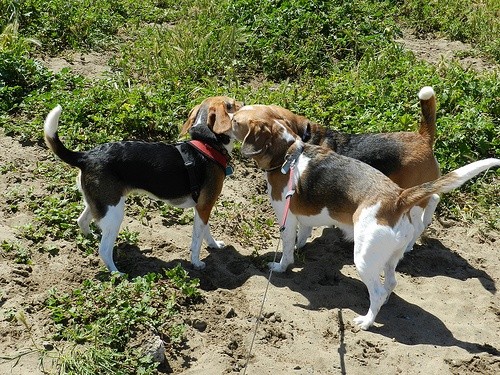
[221,108,500,330]
[228,86,443,252]
[43,96,243,280]
